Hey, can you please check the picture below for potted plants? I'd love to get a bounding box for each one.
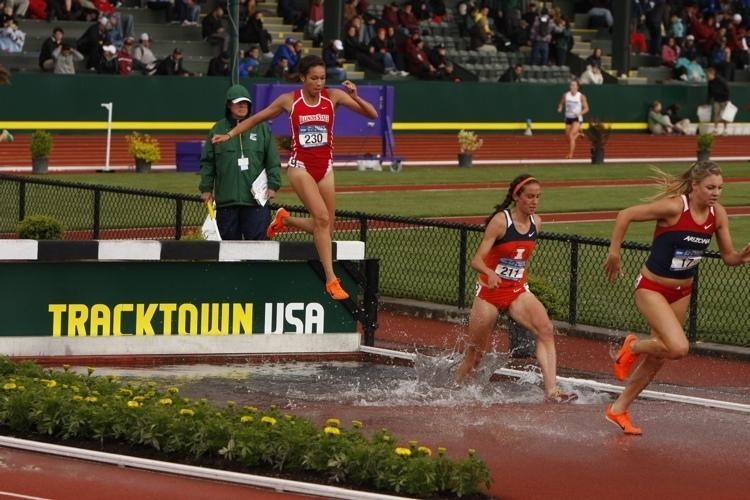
[695,130,717,162]
[456,129,483,167]
[29,130,53,174]
[125,131,161,173]
[582,118,612,164]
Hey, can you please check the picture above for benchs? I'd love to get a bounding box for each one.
[0,0,274,77]
[364,0,577,84]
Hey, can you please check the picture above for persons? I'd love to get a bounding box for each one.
[1,1,750,80]
[602,160,750,435]
[450,173,577,404]
[558,79,589,159]
[647,100,678,134]
[200,84,282,240]
[703,68,730,136]
[212,55,379,301]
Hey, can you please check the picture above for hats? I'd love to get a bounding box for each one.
[232,96,251,105]
[334,40,343,50]
[102,44,116,55]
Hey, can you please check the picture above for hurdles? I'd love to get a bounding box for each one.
[0,239,380,357]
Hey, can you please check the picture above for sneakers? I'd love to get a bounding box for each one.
[267,206,290,238]
[605,403,642,435]
[545,391,578,404]
[325,277,351,299]
[613,335,641,381]
